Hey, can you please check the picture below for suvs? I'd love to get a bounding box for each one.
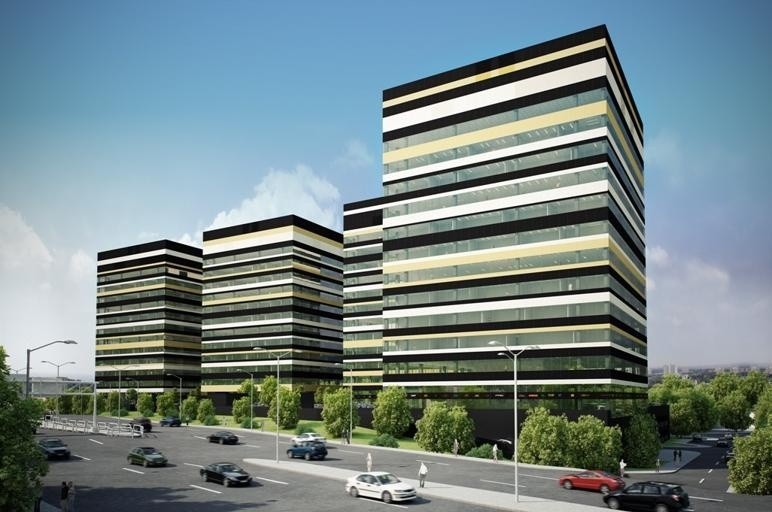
[128,417,152,432]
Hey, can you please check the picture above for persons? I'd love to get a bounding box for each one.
[673,450,678,461]
[679,450,682,462]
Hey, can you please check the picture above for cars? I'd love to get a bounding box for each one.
[286,440,328,461]
[159,417,182,427]
[38,438,72,460]
[293,433,326,445]
[693,431,737,464]
[127,446,168,467]
[345,470,416,503]
[603,480,689,511]
[206,430,239,445]
[200,461,252,487]
[559,469,625,493]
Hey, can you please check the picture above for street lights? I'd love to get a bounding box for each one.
[498,352,518,462]
[167,373,182,420]
[253,346,302,465]
[488,339,541,502]
[334,362,353,445]
[26,339,78,401]
[7,359,139,433]
[237,369,253,429]
